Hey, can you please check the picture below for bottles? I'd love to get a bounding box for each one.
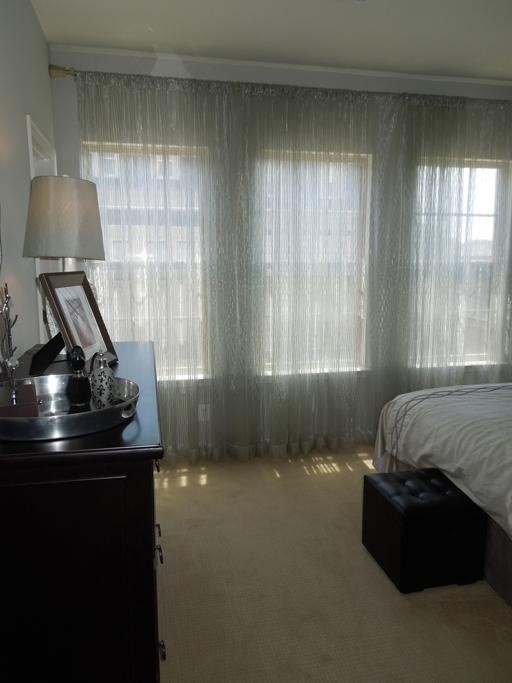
[65,349,113,406]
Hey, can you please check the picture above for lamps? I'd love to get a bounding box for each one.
[22,169,108,272]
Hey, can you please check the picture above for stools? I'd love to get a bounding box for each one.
[359,466,492,594]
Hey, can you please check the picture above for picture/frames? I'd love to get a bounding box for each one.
[28,268,119,376]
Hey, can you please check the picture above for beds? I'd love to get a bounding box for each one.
[371,385,511,607]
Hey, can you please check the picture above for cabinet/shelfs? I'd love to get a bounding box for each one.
[0,341,168,683]
